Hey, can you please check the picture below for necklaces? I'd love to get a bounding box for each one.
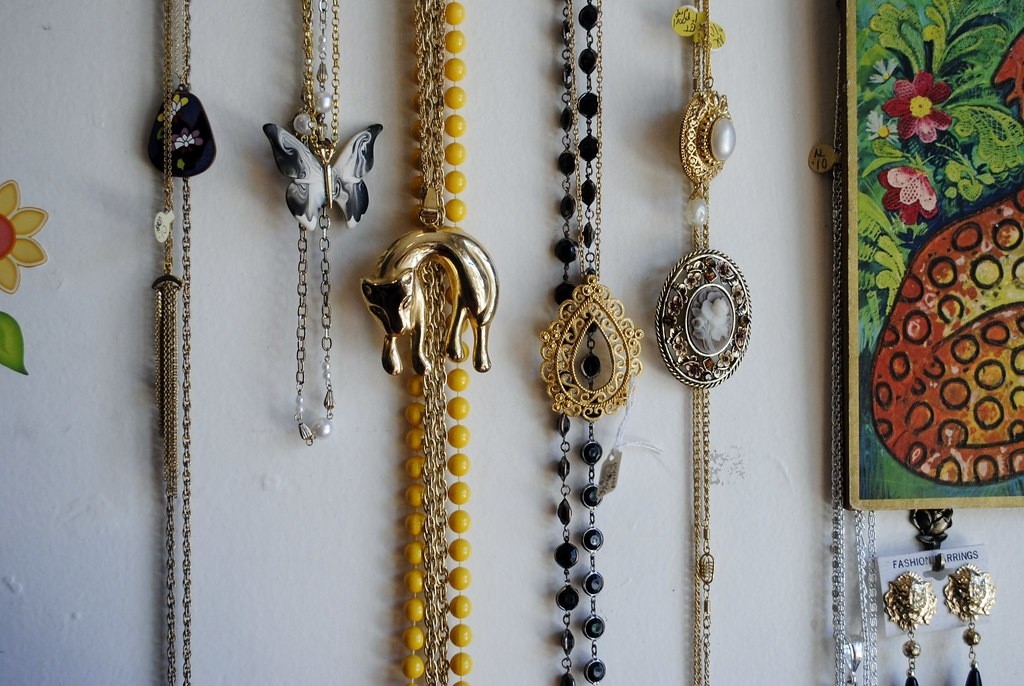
[656,0,753,686]
[359,0,500,686]
[539,1,645,686]
[263,0,382,446]
[146,0,218,686]
[809,19,878,685]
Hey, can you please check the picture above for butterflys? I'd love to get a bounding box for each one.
[262,122,384,233]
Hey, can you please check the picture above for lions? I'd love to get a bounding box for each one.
[943,564,997,622]
[883,572,938,631]
